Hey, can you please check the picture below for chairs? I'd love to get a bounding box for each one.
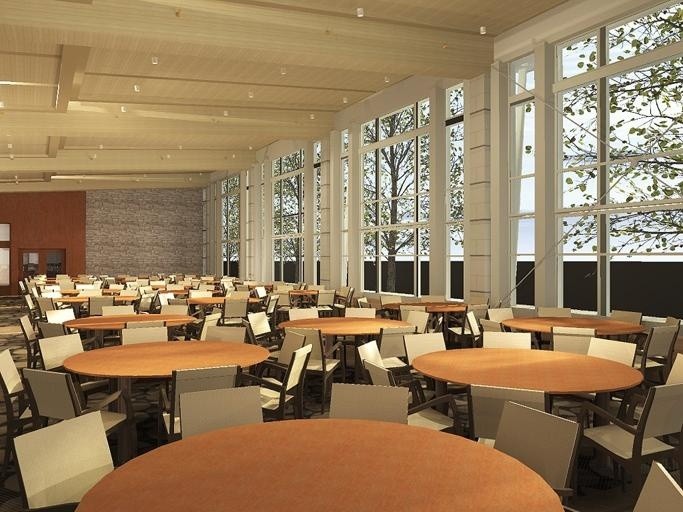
[37,332,112,406]
[102,304,136,316]
[23,294,47,331]
[586,337,638,367]
[572,383,682,500]
[258,295,280,329]
[329,383,410,425]
[38,321,65,338]
[254,331,305,382]
[245,322,284,376]
[136,275,157,313]
[335,287,354,317]
[376,325,418,359]
[157,291,175,306]
[6,408,115,512]
[204,325,247,342]
[421,295,445,304]
[448,303,487,348]
[175,311,222,340]
[402,332,448,366]
[284,327,344,416]
[536,306,572,318]
[38,274,75,299]
[318,289,336,318]
[343,307,377,320]
[75,273,138,296]
[167,298,188,305]
[465,382,546,451]
[160,306,189,316]
[120,326,169,346]
[360,301,397,321]
[307,285,325,291]
[36,296,55,322]
[216,290,250,325]
[0,348,49,468]
[289,308,319,321]
[189,289,213,299]
[466,310,482,347]
[362,359,463,435]
[609,306,644,325]
[486,307,514,322]
[17,275,38,298]
[18,314,45,370]
[20,366,136,459]
[300,283,307,289]
[87,295,115,318]
[609,321,682,402]
[634,459,683,505]
[478,318,503,332]
[157,272,188,291]
[220,275,235,296]
[550,325,597,356]
[356,296,368,308]
[663,352,683,386]
[380,295,402,306]
[247,311,271,336]
[340,287,351,298]
[492,400,583,512]
[188,274,215,289]
[124,319,165,327]
[355,339,413,386]
[234,343,313,422]
[236,277,249,290]
[406,310,430,335]
[178,384,262,441]
[483,330,532,350]
[45,307,76,325]
